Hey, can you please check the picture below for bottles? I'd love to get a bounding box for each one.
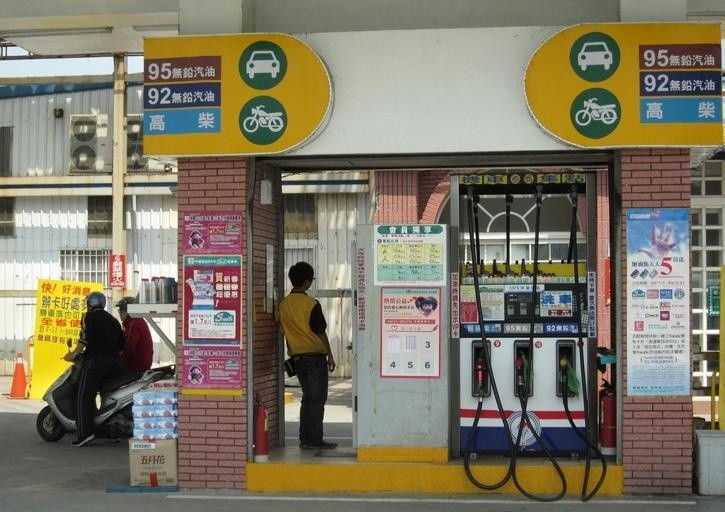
[138,277,176,304]
[134,398,178,440]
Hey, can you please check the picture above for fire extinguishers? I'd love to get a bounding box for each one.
[253,392,269,463]
[599,378,616,455]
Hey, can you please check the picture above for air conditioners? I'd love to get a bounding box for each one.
[67,114,110,175]
[126,113,149,172]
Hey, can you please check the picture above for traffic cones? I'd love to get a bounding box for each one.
[7,352,28,400]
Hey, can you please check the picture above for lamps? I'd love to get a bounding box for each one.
[54,108,64,118]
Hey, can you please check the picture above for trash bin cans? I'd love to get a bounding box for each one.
[694,430,725,495]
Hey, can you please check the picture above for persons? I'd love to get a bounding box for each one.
[116,298,154,378]
[62,291,121,448]
[420,299,433,315]
[274,261,339,450]
[638,222,681,258]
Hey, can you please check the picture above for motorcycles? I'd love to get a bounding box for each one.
[36,337,176,444]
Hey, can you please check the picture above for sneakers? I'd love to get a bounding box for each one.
[298,440,339,450]
[71,432,96,447]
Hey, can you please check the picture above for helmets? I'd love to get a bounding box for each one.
[115,296,139,309]
[86,291,106,308]
